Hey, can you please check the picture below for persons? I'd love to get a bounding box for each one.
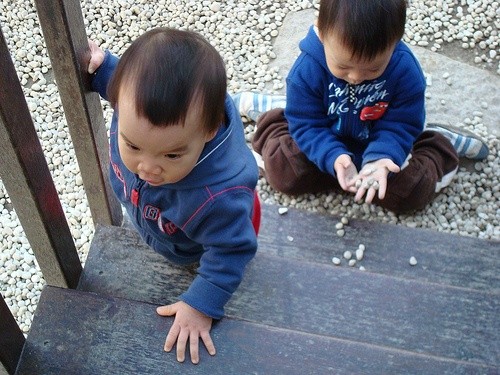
[232,0,489,214]
[85,27,262,364]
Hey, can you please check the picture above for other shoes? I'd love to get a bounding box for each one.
[231,91,288,124]
[426,123,489,161]
